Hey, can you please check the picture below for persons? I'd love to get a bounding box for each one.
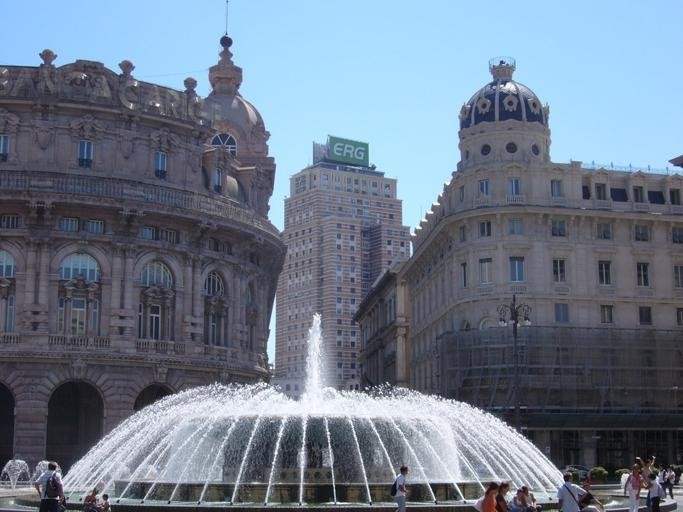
[556,471,587,512]
[522,486,543,512]
[482,481,498,512]
[394,466,410,511]
[495,482,511,512]
[623,455,675,512]
[34,461,67,512]
[81,487,101,512]
[507,489,527,511]
[96,494,112,512]
[581,482,606,512]
[577,493,601,512]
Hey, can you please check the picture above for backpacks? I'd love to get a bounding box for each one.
[44,470,59,497]
[657,483,663,497]
[390,474,402,497]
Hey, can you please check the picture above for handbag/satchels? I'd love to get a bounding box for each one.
[472,493,486,512]
[625,481,634,491]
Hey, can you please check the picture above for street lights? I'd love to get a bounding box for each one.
[497,294,532,433]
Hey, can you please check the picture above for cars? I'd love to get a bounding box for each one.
[559,465,589,480]
